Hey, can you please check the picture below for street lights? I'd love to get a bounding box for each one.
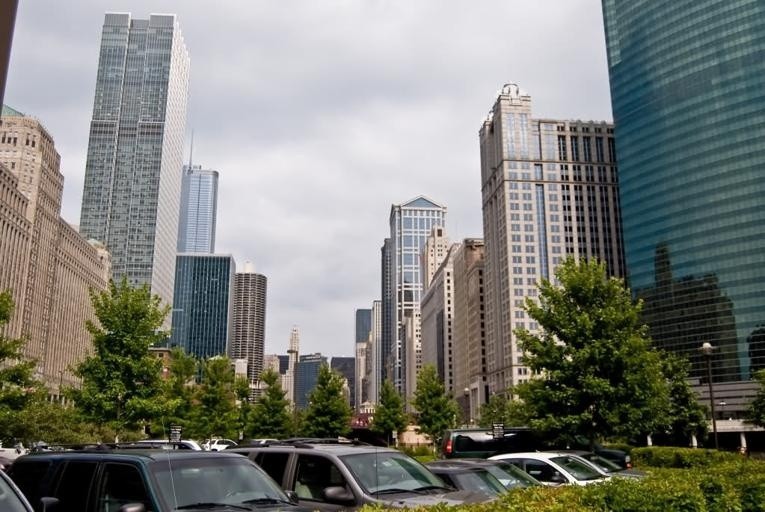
[696,340,721,449]
[463,387,471,429]
